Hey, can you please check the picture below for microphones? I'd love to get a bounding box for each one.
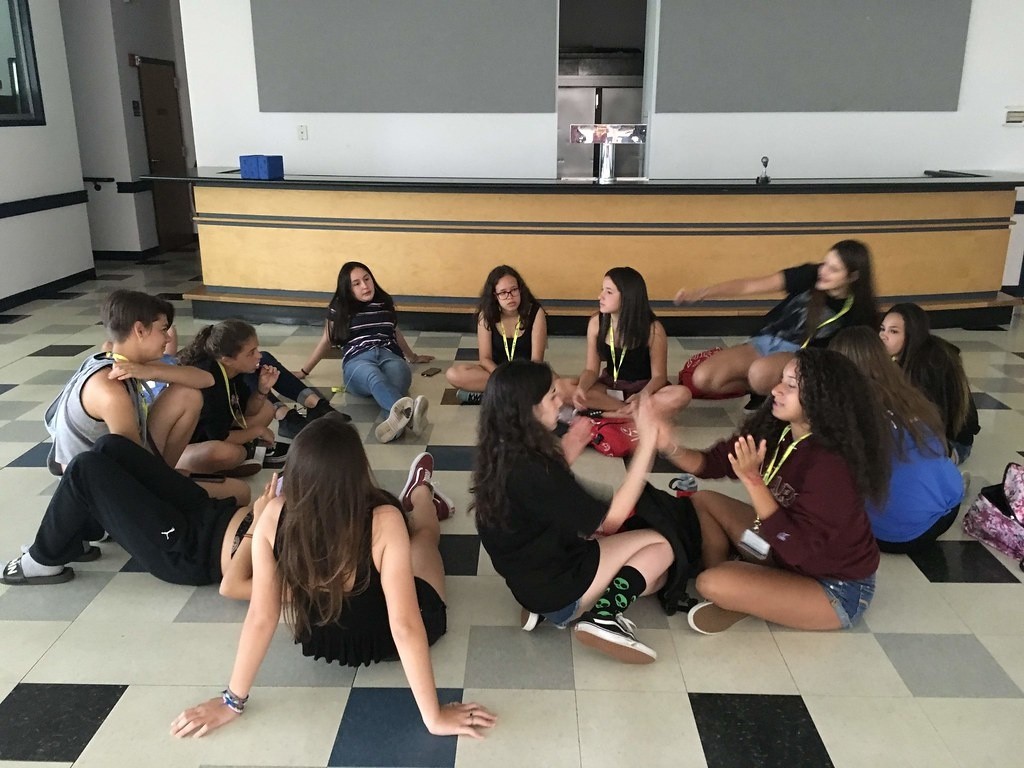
[761,157,769,167]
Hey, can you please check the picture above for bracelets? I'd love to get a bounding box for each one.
[301,368,309,376]
[243,534,254,538]
[221,686,249,714]
[658,441,677,460]
[407,353,418,363]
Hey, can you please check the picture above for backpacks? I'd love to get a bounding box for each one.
[961,462,1024,570]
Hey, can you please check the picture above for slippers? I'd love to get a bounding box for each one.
[0,557,75,585]
[23,545,101,561]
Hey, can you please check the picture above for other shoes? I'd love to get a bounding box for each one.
[687,601,752,634]
[742,393,766,415]
[571,407,604,419]
[960,470,972,491]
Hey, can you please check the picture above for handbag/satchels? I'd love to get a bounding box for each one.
[679,346,724,399]
[591,416,640,457]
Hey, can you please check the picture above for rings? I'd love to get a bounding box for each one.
[469,709,473,716]
[470,717,474,725]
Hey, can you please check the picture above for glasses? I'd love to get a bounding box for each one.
[493,287,521,299]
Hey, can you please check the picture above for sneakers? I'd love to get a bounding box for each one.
[306,398,352,422]
[398,452,434,511]
[375,396,414,444]
[278,405,309,438]
[245,439,291,463]
[406,395,429,437]
[215,460,262,477]
[431,482,455,521]
[574,612,657,664]
[456,389,485,404]
[521,606,547,631]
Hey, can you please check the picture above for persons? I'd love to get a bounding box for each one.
[287,261,436,442]
[0,434,410,600]
[829,303,982,555]
[466,360,674,664]
[446,265,561,405]
[674,240,874,415]
[555,266,693,425]
[45,290,252,542]
[653,350,909,636]
[102,318,352,481]
[170,413,499,740]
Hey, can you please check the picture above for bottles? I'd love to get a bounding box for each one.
[669,472,698,498]
